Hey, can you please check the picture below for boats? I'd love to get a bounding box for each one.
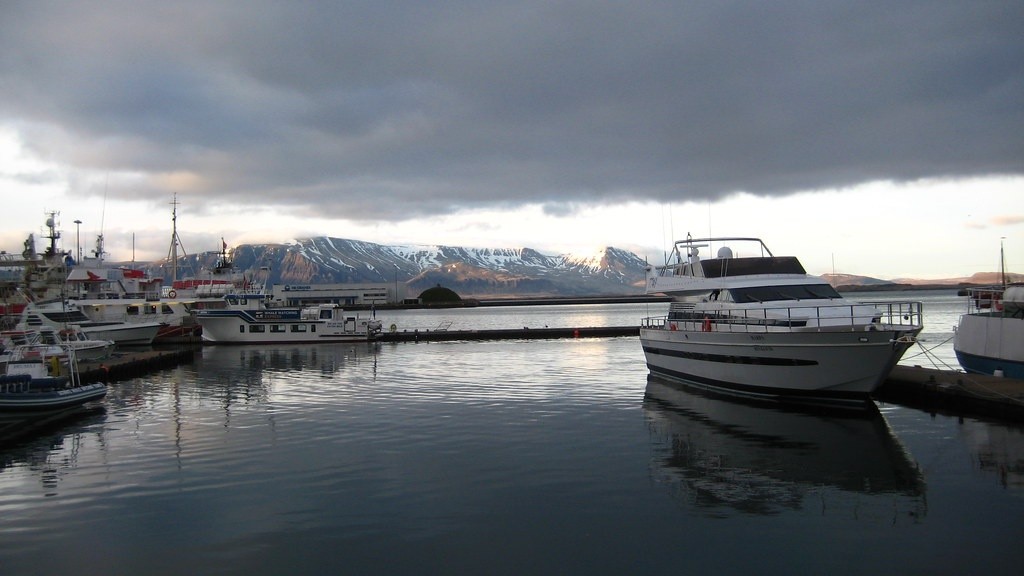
[953,238,1024,377]
[640,202,924,404]
[0,172,383,422]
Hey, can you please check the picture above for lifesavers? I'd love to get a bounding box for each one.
[995,288,1007,310]
[702,316,710,332]
[169,290,176,298]
[391,324,396,331]
